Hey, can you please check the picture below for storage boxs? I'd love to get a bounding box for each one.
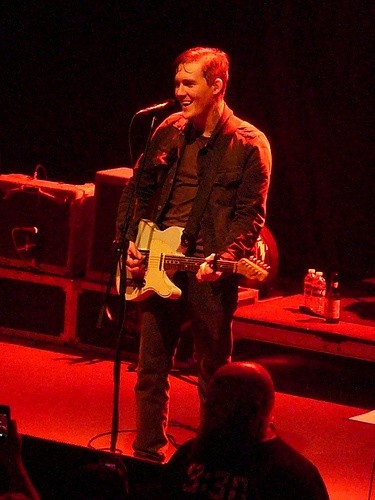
[0,175,258,371]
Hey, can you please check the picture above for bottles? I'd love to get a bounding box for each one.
[303,269,315,309]
[309,272,326,315]
[324,272,341,324]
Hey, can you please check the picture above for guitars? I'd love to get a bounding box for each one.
[116,218,272,302]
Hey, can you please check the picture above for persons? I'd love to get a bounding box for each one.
[160,362,328,500]
[116,47,273,464]
[0,404,41,500]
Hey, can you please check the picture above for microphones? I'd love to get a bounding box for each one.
[16,226,38,234]
[134,98,178,116]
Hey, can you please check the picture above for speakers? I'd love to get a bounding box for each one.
[0,174,95,278]
[95,167,135,273]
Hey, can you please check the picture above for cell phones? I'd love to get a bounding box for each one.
[0,405,11,437]
